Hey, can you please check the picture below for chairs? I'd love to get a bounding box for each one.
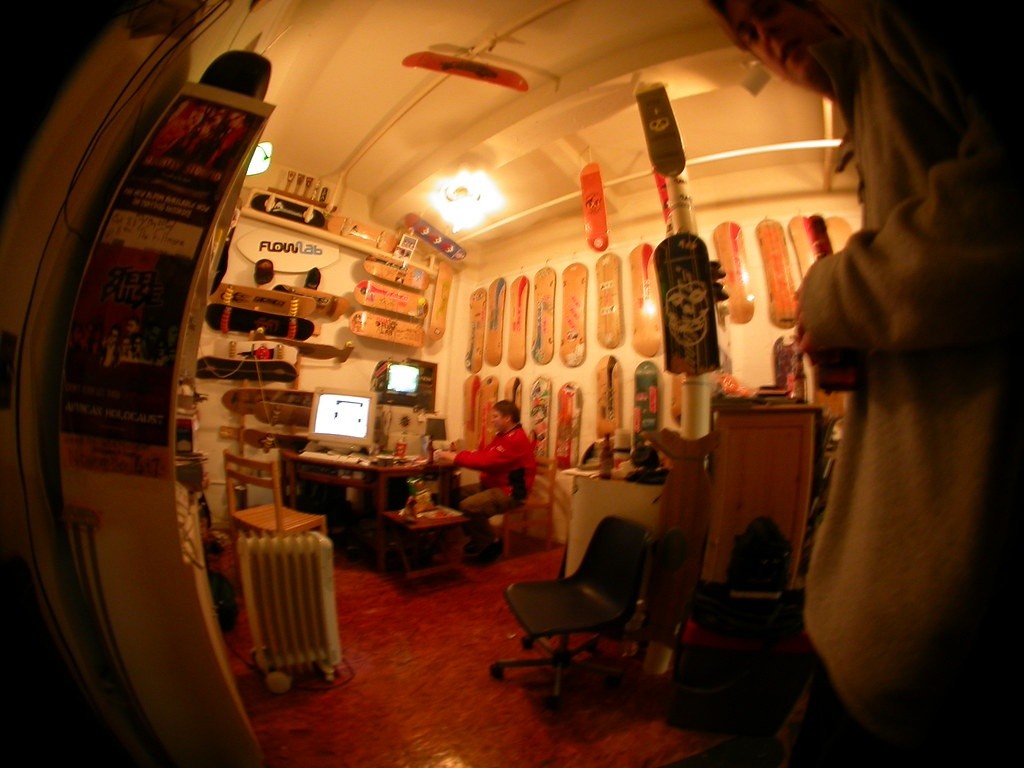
[220,448,328,545]
[491,514,651,713]
[489,455,555,553]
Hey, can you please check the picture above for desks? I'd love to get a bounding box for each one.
[283,440,460,572]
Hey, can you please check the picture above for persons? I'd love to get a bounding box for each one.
[434,400,535,567]
[706,0,1024,768]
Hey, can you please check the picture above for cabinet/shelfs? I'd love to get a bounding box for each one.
[563,461,664,606]
[60,89,277,764]
[691,399,827,639]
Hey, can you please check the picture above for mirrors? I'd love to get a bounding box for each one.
[1,3,1003,768]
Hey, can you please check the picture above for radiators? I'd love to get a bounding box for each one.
[235,530,341,671]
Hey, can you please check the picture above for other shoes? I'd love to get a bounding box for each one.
[478,541,502,561]
[465,540,479,553]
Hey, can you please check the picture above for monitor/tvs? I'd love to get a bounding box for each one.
[308,387,379,452]
[388,363,420,394]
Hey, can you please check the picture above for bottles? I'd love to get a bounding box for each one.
[393,435,407,458]
[598,432,614,481]
[790,351,807,403]
[427,435,435,463]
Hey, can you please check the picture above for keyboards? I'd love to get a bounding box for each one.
[299,451,361,465]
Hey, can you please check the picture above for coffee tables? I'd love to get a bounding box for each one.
[382,501,469,582]
